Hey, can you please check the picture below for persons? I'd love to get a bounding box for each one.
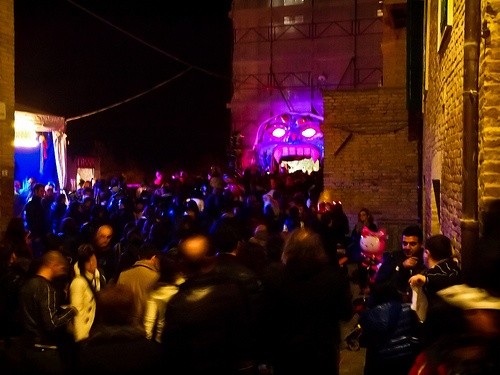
[353,208,378,253]
[408,234,463,332]
[4,169,352,374]
[374,227,425,303]
[410,199,500,375]
[360,281,423,375]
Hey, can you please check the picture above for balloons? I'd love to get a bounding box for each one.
[359,226,388,267]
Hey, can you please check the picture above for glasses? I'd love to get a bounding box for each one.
[135,207,144,212]
